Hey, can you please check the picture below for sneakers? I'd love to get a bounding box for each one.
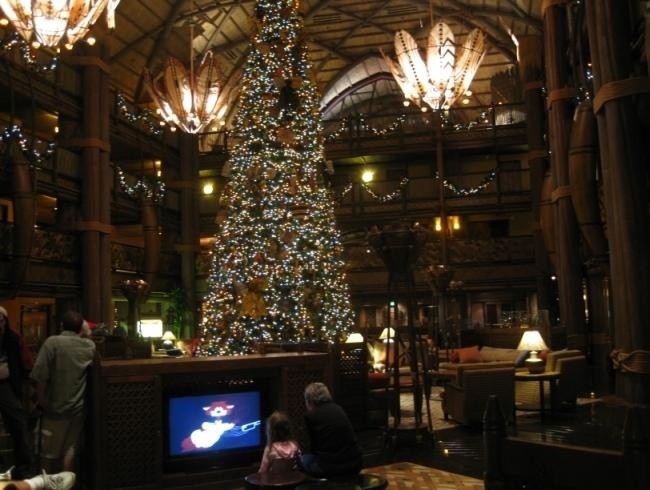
[38,468,76,490]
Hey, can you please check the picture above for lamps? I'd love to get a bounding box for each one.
[1,0,123,54]
[143,22,232,136]
[379,0,487,115]
[517,330,550,373]
[138,318,164,353]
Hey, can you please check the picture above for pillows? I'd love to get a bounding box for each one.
[454,347,548,363]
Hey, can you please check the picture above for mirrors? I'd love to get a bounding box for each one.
[20,303,50,338]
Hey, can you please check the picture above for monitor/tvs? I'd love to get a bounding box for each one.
[163,391,266,469]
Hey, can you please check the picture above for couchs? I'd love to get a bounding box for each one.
[438,346,586,411]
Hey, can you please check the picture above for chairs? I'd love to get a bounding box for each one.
[575,348,650,451]
[442,364,516,424]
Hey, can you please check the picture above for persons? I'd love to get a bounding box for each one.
[300,381,366,479]
[256,411,302,473]
[0,464,78,490]
[1,307,27,462]
[27,311,98,476]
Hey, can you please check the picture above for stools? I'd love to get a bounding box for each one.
[295,473,388,490]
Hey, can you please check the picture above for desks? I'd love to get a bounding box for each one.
[514,370,563,423]
[244,471,306,490]
